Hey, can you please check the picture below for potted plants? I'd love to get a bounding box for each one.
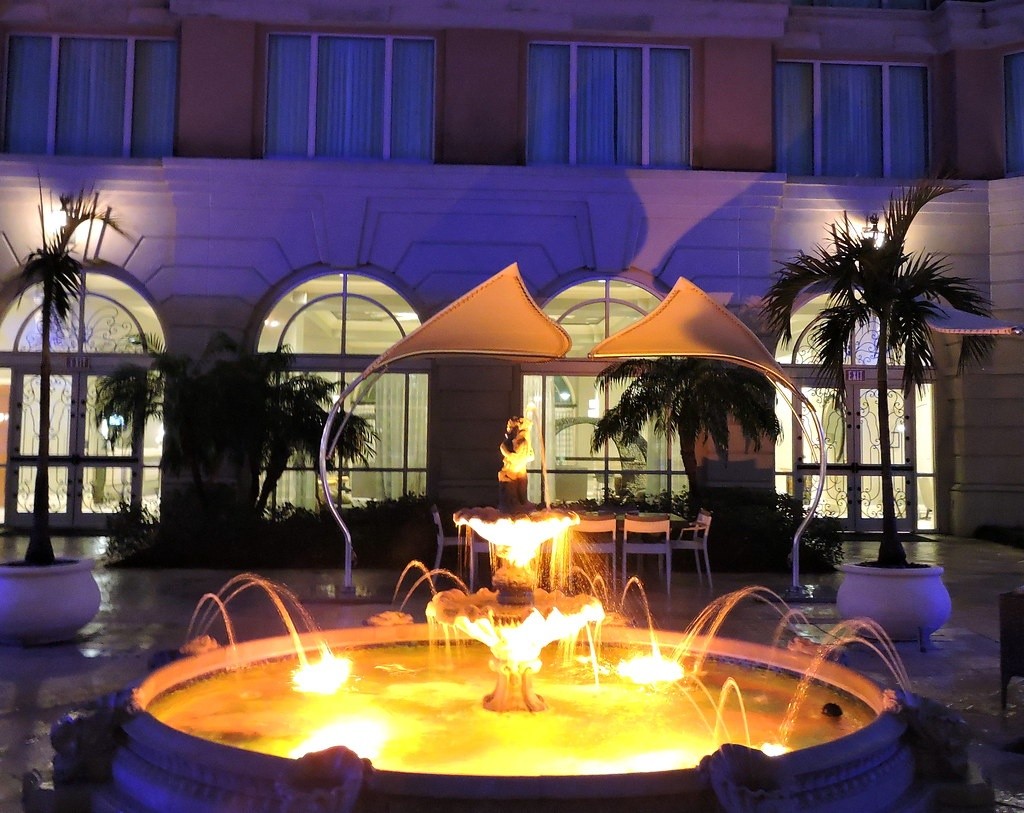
[0,189,132,643]
[761,173,999,643]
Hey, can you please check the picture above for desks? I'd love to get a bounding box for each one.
[463,513,685,594]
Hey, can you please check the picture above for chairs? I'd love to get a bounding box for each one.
[1000,586,1024,710]
[430,501,714,598]
